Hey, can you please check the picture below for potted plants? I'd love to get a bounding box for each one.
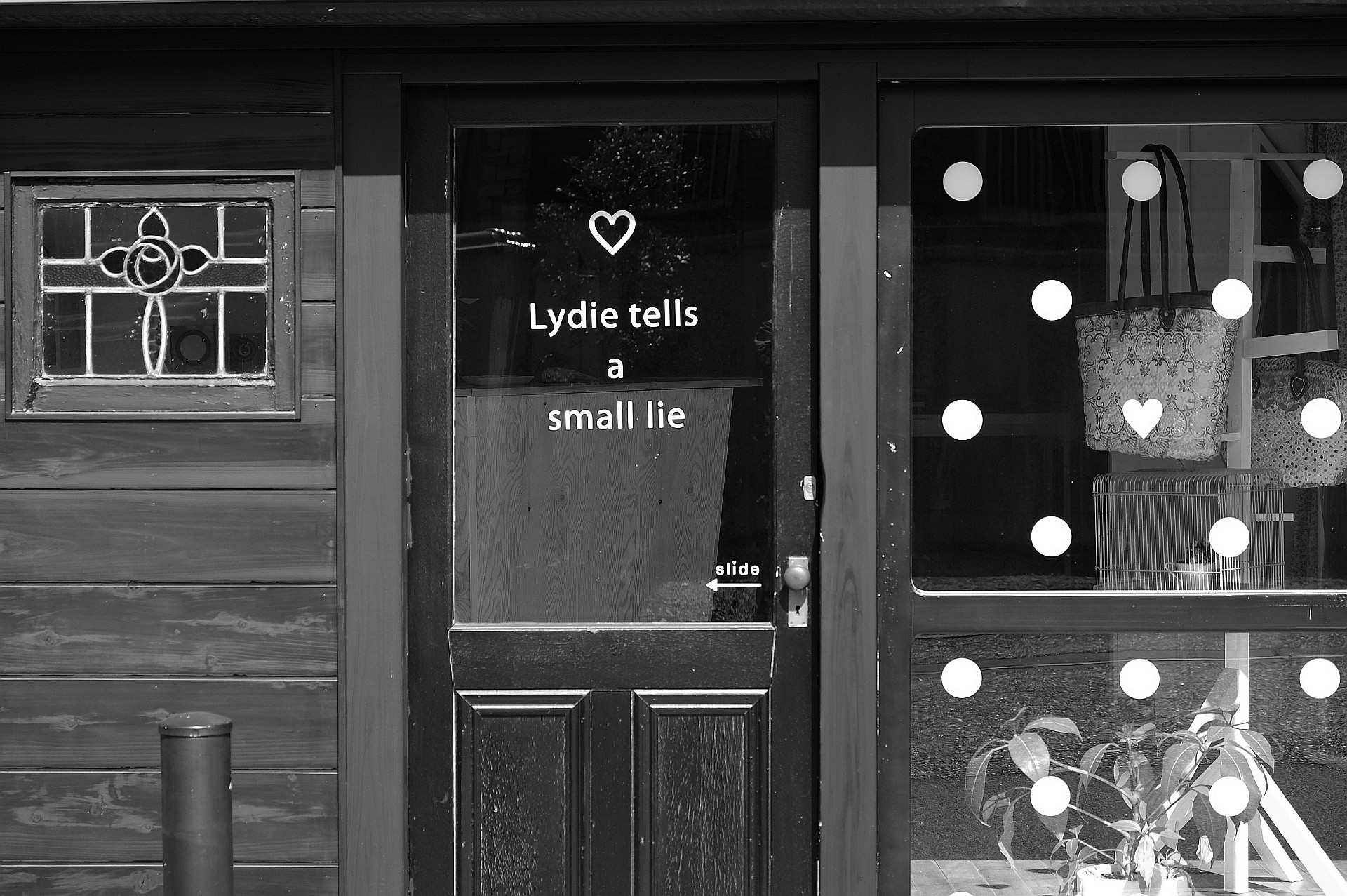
[958,693,1291,896]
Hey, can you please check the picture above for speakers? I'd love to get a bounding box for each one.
[230,333,264,372]
[169,325,217,374]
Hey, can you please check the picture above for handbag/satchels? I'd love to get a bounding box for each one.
[1250,239,1347,488]
[1072,142,1241,462]
